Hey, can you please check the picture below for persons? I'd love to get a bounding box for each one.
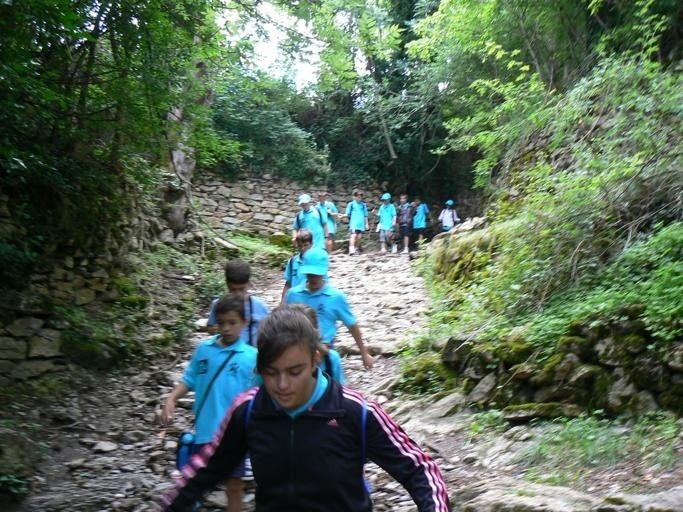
[158,295,266,512]
[278,247,377,373]
[291,303,348,388]
[279,229,313,303]
[289,187,461,258]
[138,303,453,512]
[204,256,272,351]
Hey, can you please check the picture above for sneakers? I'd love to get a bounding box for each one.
[349,247,363,255]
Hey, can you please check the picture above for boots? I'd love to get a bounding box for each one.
[378,243,409,254]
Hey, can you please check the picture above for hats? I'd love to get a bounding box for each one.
[298,247,329,277]
[445,199,454,206]
[381,192,391,200]
[298,194,310,205]
